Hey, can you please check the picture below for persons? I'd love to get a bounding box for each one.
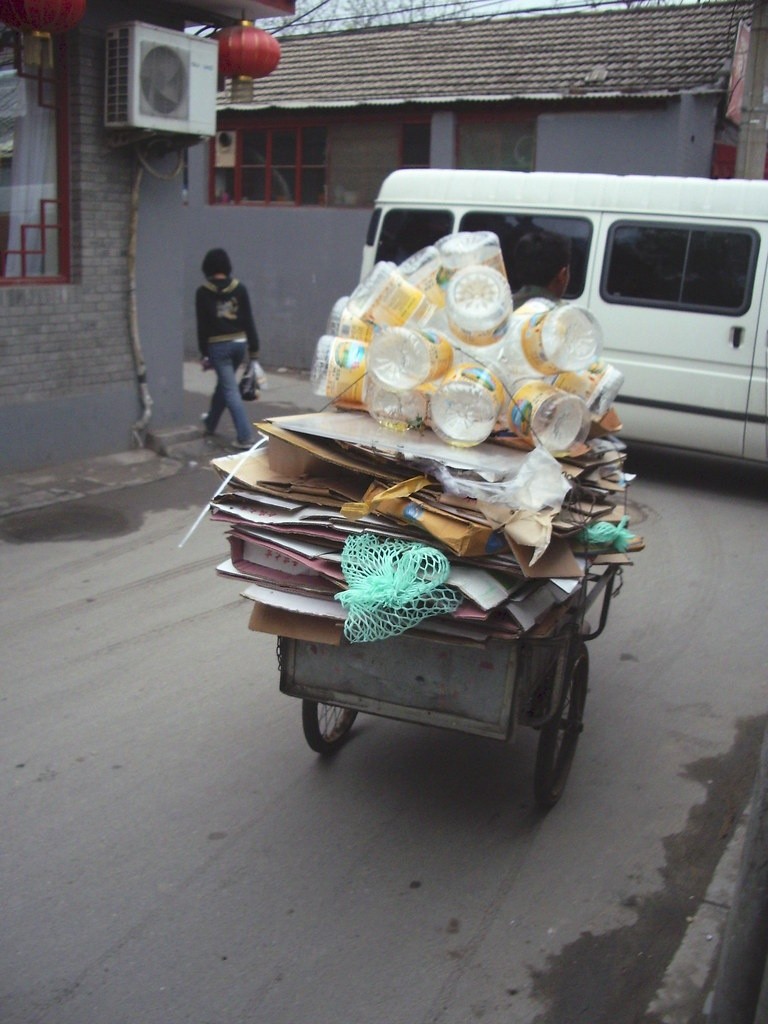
[193,247,262,449]
[509,228,571,310]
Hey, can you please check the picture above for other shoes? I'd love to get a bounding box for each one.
[231,437,254,449]
[201,413,215,434]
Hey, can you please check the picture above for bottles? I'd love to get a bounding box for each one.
[309,231,624,456]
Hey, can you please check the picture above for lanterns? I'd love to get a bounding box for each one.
[209,21,281,104]
[0,0,86,66]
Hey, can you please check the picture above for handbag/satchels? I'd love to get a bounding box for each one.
[238,357,267,401]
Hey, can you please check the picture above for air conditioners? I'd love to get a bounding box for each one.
[102,21,220,142]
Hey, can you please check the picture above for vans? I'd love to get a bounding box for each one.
[360,165,768,480]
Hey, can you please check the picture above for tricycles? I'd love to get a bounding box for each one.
[271,548,631,815]
